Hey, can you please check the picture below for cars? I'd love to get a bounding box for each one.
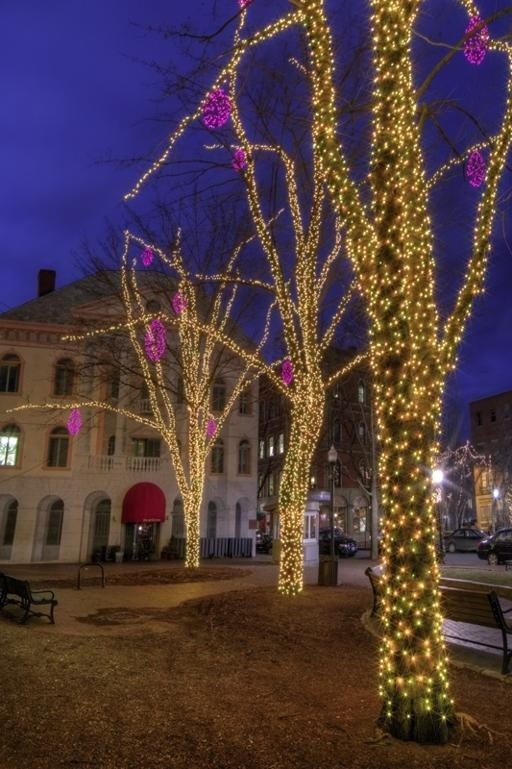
[444,528,489,553]
[477,528,512,565]
[320,528,357,558]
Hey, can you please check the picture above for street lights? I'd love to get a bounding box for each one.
[318,443,338,587]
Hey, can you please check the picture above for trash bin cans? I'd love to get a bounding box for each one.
[318,559,339,587]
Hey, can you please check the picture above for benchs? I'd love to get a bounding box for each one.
[0,571,57,626]
[365,567,512,675]
